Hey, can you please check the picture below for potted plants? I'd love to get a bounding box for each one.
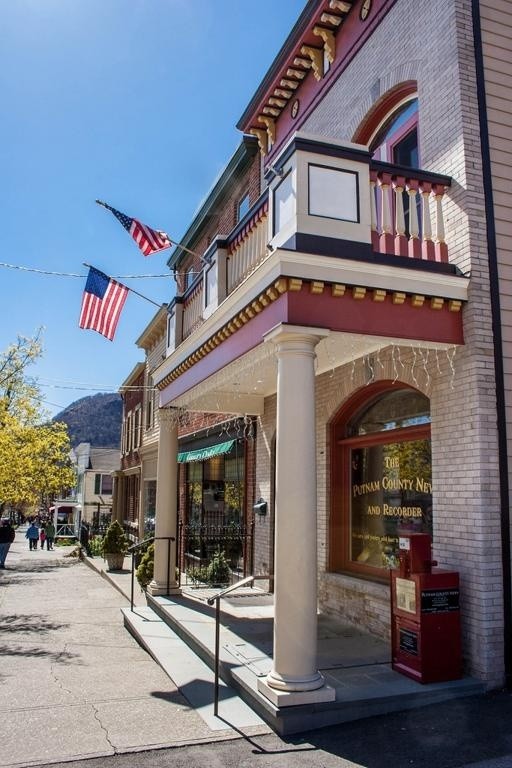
[100,521,130,570]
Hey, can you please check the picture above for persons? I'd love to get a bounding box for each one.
[39,529,45,548]
[45,520,55,551]
[26,513,33,525]
[25,522,39,551]
[23,517,26,523]
[0,518,15,568]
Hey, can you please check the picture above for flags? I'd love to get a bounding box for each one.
[78,265,129,343]
[103,204,171,256]
